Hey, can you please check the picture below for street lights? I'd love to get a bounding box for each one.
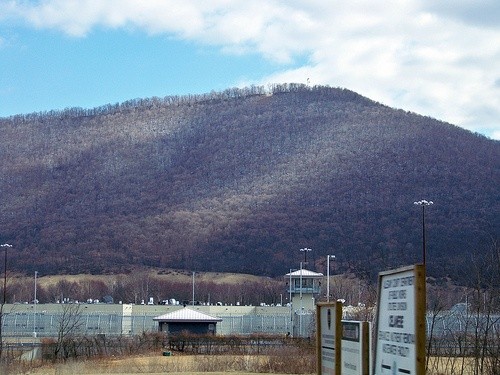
[299,247,312,269]
[413,199,434,264]
[1,244,12,304]
[327,255,336,302]
